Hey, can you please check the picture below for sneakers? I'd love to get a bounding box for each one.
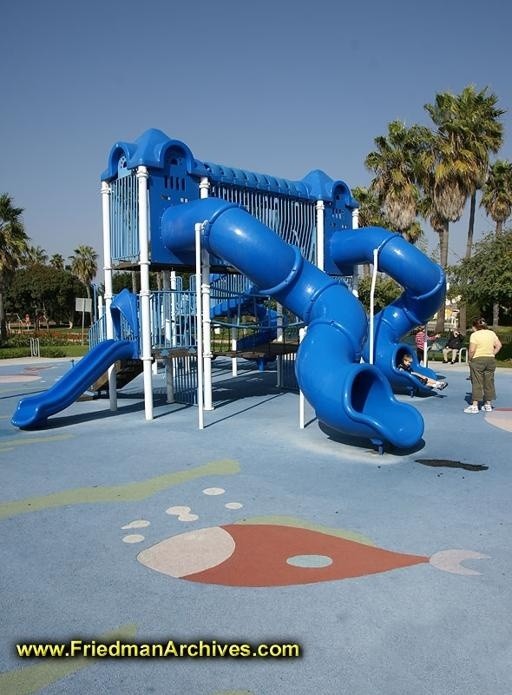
[480,404,492,412]
[463,405,479,414]
[437,382,448,390]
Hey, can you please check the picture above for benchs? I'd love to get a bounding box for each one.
[408,336,470,365]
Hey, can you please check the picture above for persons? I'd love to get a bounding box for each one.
[415,326,442,365]
[442,330,465,364]
[398,353,448,391]
[462,316,504,414]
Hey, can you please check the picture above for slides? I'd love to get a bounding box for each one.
[12,339,138,429]
[161,199,423,452]
[330,227,447,398]
